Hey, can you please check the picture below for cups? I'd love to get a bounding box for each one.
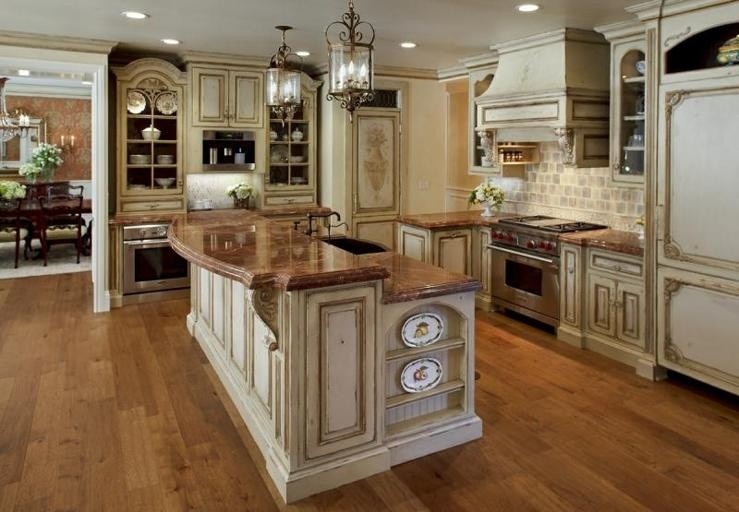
[234,153,245,164]
[481,156,492,167]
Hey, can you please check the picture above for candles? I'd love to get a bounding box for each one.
[60,135,79,155]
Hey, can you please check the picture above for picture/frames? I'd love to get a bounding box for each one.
[0,114,49,177]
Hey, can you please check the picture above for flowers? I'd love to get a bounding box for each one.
[17,143,62,182]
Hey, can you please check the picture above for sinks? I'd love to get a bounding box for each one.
[316,232,394,258]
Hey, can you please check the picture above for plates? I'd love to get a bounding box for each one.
[156,94,177,115]
[401,312,443,347]
[128,184,150,191]
[400,357,442,393]
[127,91,147,114]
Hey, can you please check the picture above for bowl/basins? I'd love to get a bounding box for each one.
[635,60,644,75]
[155,178,176,186]
[130,155,151,164]
[157,154,174,164]
[716,34,739,66]
[291,155,304,163]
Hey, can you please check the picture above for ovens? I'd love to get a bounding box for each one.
[485,240,561,337]
[123,226,192,296]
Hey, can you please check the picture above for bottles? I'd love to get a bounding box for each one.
[291,129,304,142]
[498,148,523,163]
[268,130,278,141]
[628,128,643,147]
[209,148,218,165]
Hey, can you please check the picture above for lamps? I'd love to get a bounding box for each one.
[0,76,31,142]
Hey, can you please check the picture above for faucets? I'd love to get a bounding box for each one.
[327,221,350,240]
[307,210,341,233]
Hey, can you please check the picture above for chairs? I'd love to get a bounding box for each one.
[0,178,93,270]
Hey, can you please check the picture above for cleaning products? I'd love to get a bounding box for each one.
[235,154,246,165]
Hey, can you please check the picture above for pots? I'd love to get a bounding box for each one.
[142,124,163,140]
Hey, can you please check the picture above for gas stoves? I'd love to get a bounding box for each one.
[492,213,607,257]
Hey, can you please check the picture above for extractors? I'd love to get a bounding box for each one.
[477,28,611,170]
